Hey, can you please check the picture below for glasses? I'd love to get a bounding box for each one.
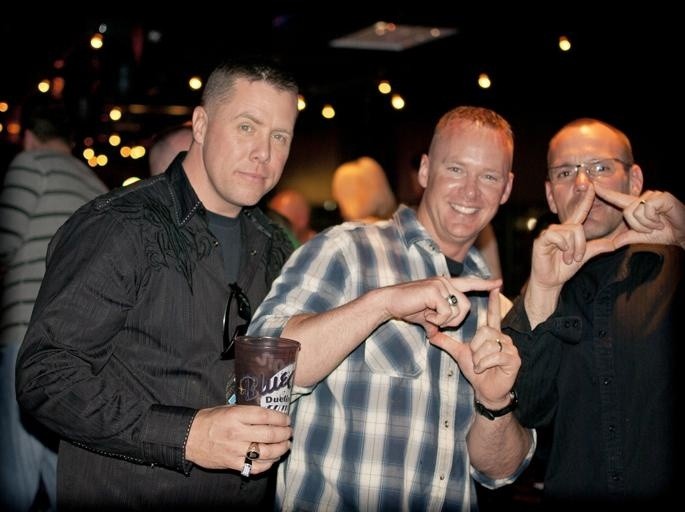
[218,284,251,360]
[547,157,631,185]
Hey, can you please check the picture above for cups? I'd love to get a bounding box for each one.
[233,335,300,419]
